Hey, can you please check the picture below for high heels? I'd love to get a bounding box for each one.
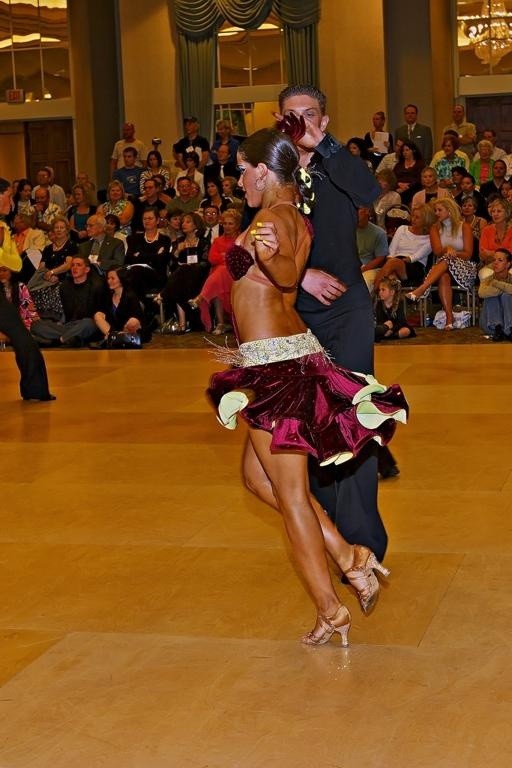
[300,605,352,648]
[342,544,392,615]
[444,317,455,331]
[405,289,425,303]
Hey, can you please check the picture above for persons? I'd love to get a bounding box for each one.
[204,128,391,648]
[347,103,511,342]
[1,117,257,401]
[272,80,388,586]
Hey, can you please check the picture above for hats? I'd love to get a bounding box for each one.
[183,116,197,123]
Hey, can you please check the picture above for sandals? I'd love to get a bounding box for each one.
[212,322,225,334]
[187,298,200,309]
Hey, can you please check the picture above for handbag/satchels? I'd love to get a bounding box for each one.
[432,304,472,330]
[106,328,142,348]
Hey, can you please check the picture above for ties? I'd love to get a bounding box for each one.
[409,125,414,143]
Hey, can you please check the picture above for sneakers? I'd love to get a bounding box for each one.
[66,335,81,346]
[492,330,505,341]
[374,325,385,343]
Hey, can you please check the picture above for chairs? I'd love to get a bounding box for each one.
[472,283,481,327]
[423,283,471,328]
[401,268,424,327]
[146,245,173,325]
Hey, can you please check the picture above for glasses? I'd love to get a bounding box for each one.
[156,182,165,187]
[177,184,189,188]
[206,212,216,216]
[143,186,153,190]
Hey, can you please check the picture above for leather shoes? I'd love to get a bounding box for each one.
[23,392,56,401]
[380,464,400,479]
